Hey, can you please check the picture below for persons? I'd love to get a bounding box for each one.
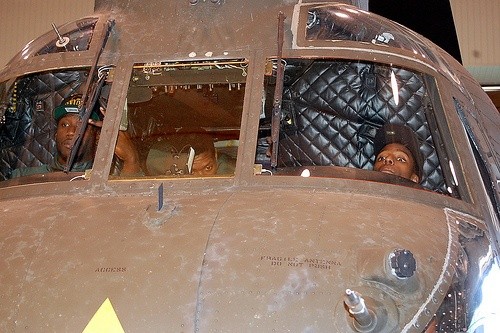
[169,126,237,177]
[10,93,148,178]
[371,122,424,187]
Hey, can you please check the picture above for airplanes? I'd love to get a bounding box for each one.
[2,0,499,333]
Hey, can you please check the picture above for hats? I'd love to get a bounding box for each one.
[374,121,424,181]
[53,93,103,121]
